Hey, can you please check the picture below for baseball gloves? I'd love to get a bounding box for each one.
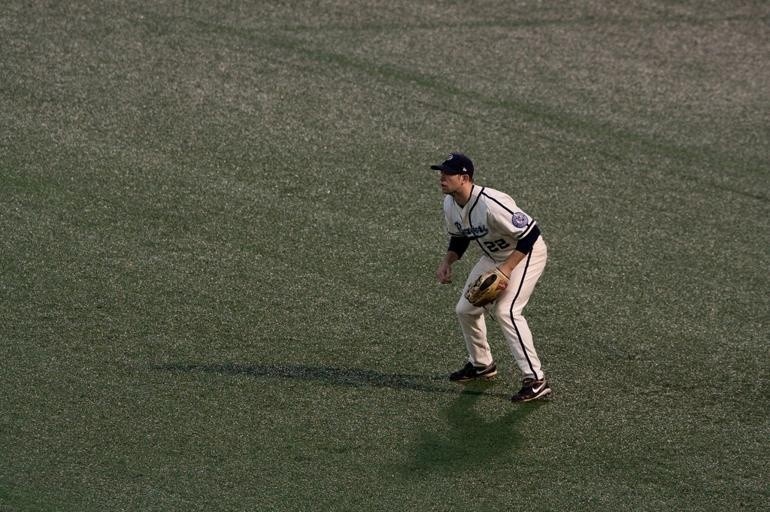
[464,267,511,307]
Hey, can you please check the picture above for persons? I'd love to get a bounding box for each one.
[430,151,554,405]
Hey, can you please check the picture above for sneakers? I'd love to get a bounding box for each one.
[511,378,552,403]
[449,360,498,381]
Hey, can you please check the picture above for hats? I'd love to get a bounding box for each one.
[430,152,474,176]
